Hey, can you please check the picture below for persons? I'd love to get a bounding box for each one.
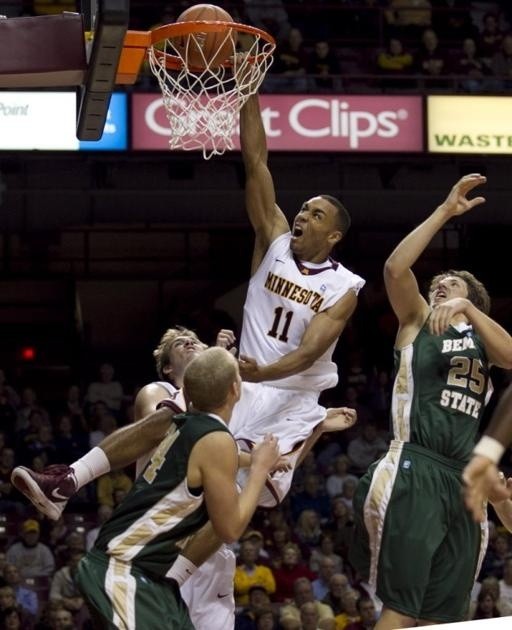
[353,171,512,629]
[1,330,512,630]
[251,0,512,88]
[12,50,367,584]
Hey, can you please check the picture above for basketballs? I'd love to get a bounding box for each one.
[173,5,237,69]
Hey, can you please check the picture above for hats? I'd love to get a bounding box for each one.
[24,520,39,533]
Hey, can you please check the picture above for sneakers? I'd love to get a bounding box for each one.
[10,465,75,522]
